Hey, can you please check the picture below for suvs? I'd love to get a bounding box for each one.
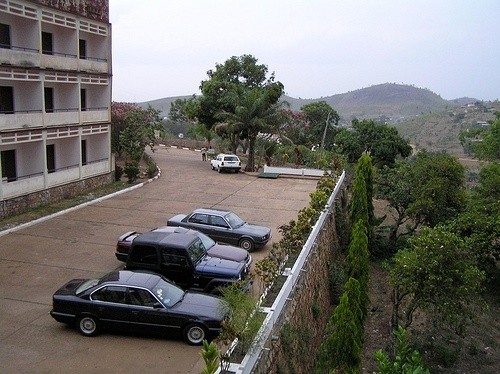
[124,231,254,298]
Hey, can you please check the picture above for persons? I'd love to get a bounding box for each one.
[200,146,213,161]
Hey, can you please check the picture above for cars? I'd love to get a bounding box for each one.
[115,225,253,271]
[166,208,272,253]
[49,271,234,345]
[210,152,242,173]
